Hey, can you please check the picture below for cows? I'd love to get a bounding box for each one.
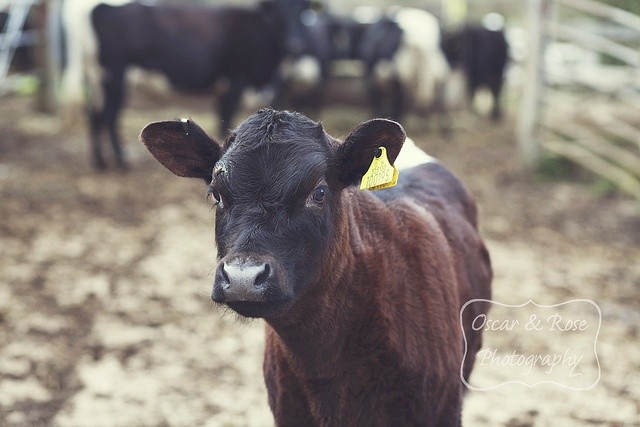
[85,1,319,180]
[139,104,518,427]
